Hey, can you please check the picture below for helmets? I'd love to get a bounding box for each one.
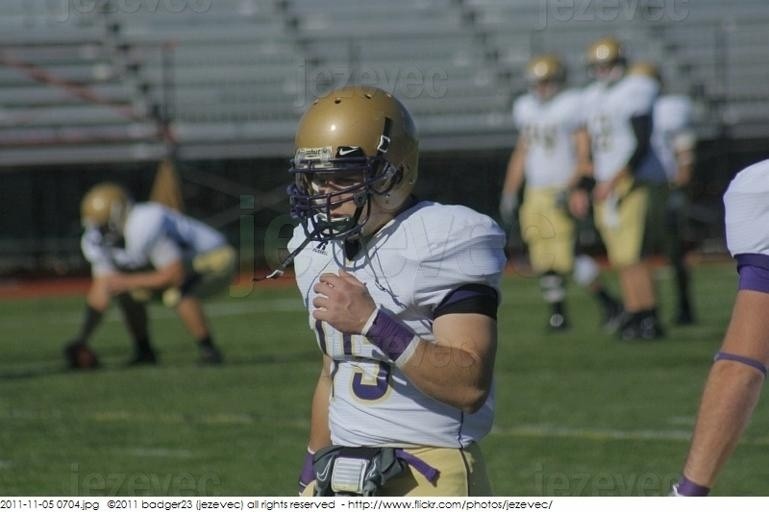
[586,38,625,69]
[79,182,132,249]
[626,62,660,83]
[525,56,565,84]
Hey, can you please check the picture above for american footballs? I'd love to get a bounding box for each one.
[64,342,100,367]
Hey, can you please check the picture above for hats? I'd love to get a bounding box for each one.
[287,85,419,243]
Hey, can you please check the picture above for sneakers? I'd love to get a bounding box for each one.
[124,347,158,367]
[195,347,225,366]
[673,308,695,327]
[547,313,569,331]
[595,303,661,342]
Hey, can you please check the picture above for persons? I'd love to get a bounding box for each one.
[64,180,238,368]
[501,56,582,331]
[567,37,664,343]
[573,136,625,329]
[284,86,507,497]
[665,158,769,496]
[627,54,702,326]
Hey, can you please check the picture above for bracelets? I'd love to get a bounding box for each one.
[361,306,421,370]
[574,173,596,193]
